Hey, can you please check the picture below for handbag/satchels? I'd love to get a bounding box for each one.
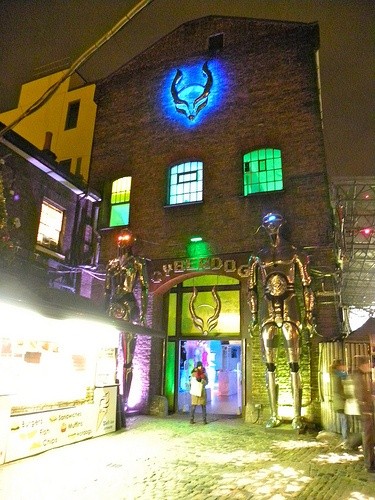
[190,381,203,397]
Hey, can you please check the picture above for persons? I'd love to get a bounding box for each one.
[327,357,357,454]
[246,212,321,430]
[187,361,211,425]
[345,357,375,473]
[102,229,151,413]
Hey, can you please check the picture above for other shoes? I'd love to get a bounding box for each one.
[203,420,207,424]
[190,419,193,424]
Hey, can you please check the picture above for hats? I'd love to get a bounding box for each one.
[197,361,202,367]
